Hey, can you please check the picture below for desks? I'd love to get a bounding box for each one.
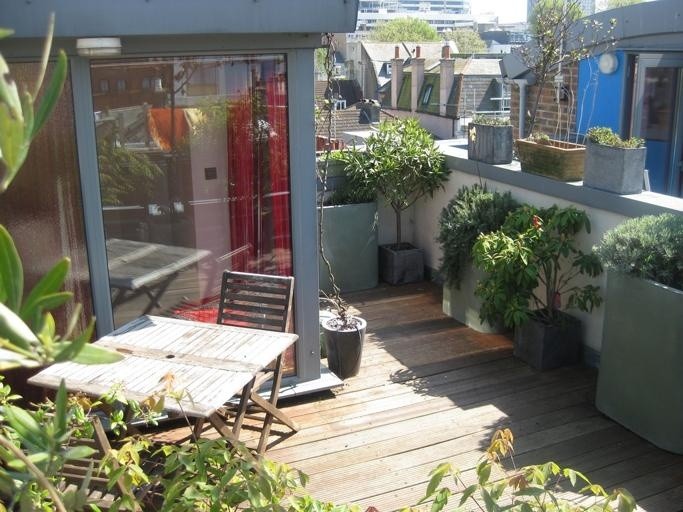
[107,236,209,317]
[25,314,299,467]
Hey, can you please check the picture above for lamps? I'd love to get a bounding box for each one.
[75,36,123,58]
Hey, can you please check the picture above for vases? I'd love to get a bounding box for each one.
[515,304,581,370]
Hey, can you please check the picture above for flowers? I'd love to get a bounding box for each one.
[472,202,602,330]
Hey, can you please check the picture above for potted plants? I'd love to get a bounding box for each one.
[584,127,647,193]
[511,133,587,183]
[437,181,514,334]
[593,214,683,457]
[335,117,443,283]
[468,115,513,164]
[319,33,367,378]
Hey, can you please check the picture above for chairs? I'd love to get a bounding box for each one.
[181,271,299,474]
